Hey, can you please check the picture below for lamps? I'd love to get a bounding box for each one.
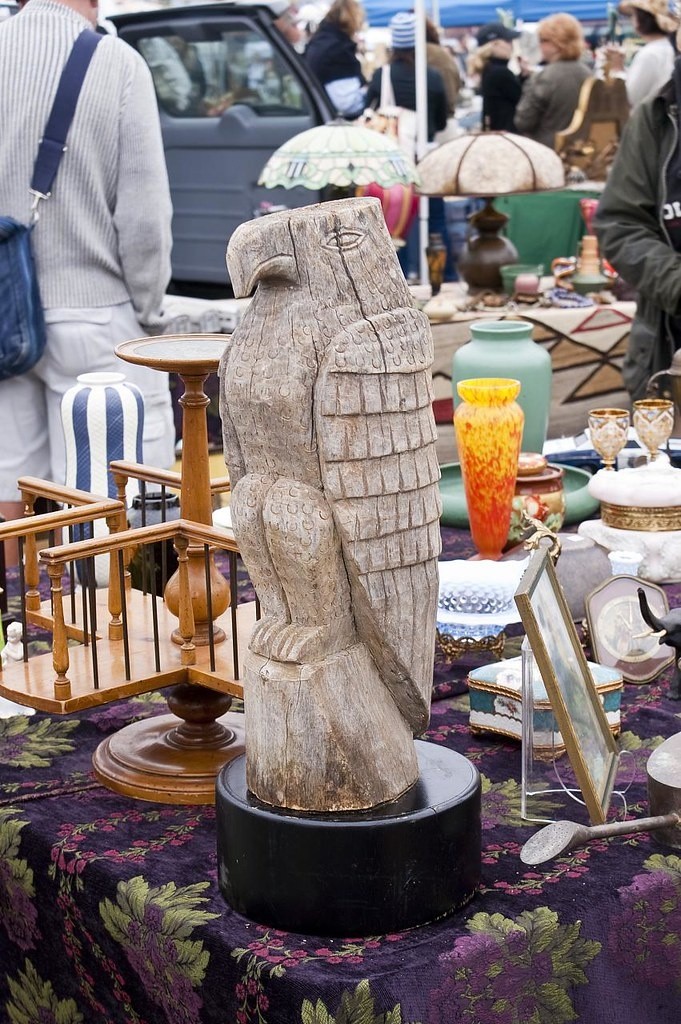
[420,116,565,298]
[257,118,416,213]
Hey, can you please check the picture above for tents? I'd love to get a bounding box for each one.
[363,0,673,27]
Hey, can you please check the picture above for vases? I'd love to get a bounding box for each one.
[455,379,524,563]
[450,319,551,459]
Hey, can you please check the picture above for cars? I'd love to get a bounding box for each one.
[0,0,350,308]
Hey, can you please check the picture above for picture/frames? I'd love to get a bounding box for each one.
[513,548,618,826]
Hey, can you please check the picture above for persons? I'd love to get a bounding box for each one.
[140,0,681,150]
[0,0,174,574]
[591,21,681,402]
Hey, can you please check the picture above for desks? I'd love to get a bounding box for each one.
[424,300,638,435]
[496,182,609,276]
[0,458,681,1024]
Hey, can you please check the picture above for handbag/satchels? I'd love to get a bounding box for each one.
[0,212,47,381]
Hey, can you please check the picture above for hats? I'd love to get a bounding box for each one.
[616,1,678,32]
[389,15,417,47]
[477,23,522,45]
